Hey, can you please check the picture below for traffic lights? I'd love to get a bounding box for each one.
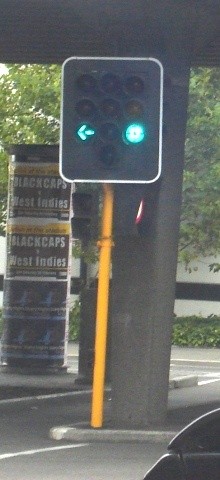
[114,190,147,244]
[70,189,99,242]
[58,56,164,184]
